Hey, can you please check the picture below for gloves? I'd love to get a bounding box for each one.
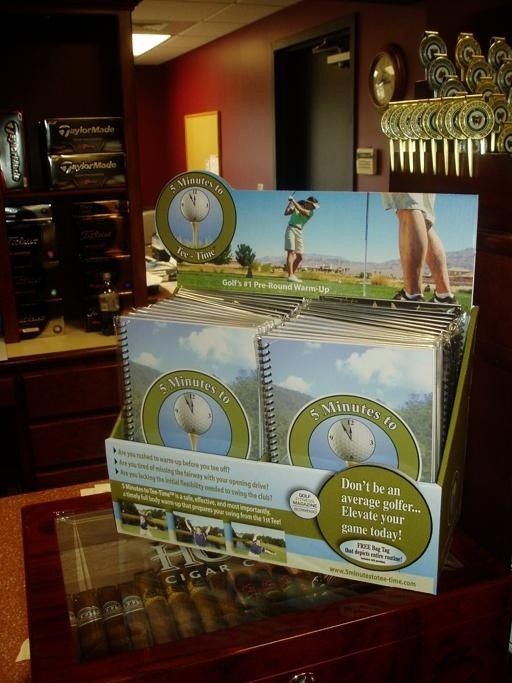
[288,195,293,201]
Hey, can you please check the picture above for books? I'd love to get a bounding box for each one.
[112,287,309,462]
[258,296,464,483]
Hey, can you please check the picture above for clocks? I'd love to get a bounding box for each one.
[365,48,410,116]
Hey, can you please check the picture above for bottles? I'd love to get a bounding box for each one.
[99,272,120,336]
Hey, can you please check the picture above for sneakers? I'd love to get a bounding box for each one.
[372,289,426,311]
[427,290,457,312]
[288,275,301,282]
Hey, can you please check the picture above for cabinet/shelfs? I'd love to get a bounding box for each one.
[0,348,128,485]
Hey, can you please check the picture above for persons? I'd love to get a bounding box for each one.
[248,533,276,559]
[381,193,458,304]
[284,196,320,282]
[184,518,213,546]
[139,515,157,537]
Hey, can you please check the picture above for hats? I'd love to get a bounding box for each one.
[307,199,319,209]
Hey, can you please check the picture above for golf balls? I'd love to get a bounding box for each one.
[173,392,213,435]
[180,189,210,221]
[338,281,342,284]
[327,418,376,463]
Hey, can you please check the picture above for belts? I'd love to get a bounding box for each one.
[289,224,302,231]
